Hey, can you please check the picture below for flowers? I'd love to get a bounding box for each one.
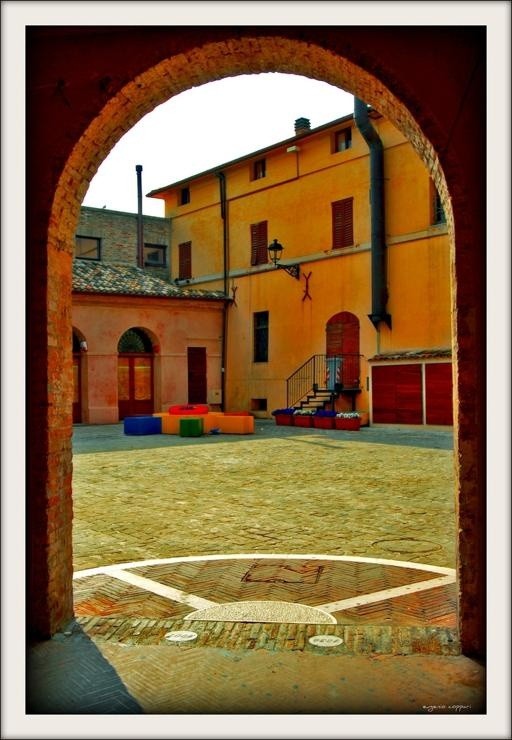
[313,410,336,417]
[335,411,360,419]
[270,407,294,416]
[293,409,316,417]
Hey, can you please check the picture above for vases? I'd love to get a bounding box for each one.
[334,417,361,431]
[275,414,293,426]
[312,416,335,429]
[293,414,312,428]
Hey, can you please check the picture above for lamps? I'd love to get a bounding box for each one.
[266,238,299,280]
